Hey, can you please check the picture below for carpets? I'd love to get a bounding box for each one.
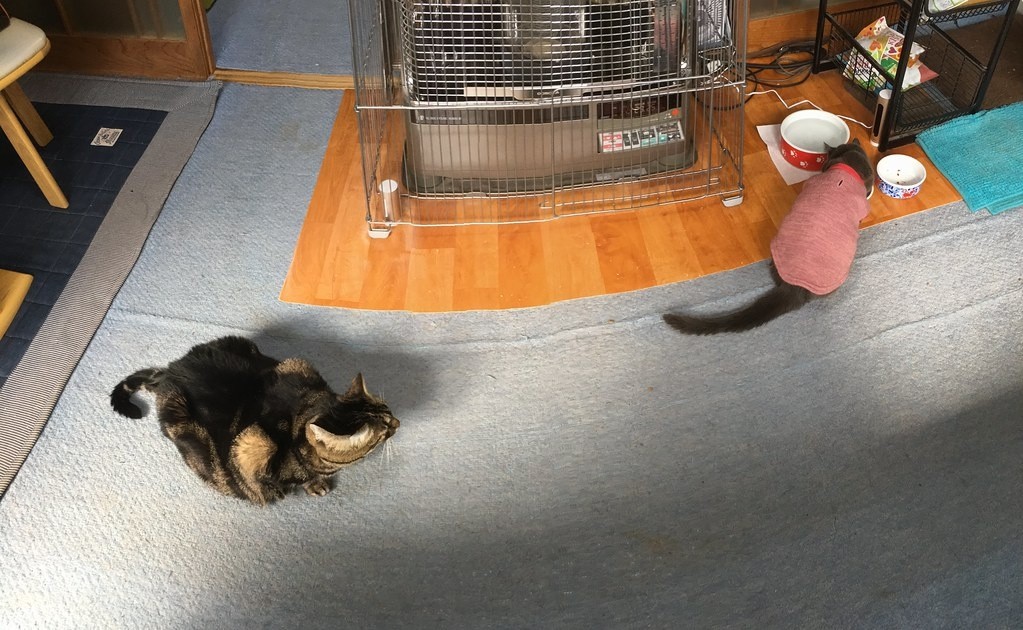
[0,73,224,499]
[916,101,1023,215]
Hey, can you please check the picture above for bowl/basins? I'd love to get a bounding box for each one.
[780,110,851,171]
[877,154,927,198]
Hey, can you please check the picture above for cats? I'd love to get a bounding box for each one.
[110,337,400,505]
[663,136,875,335]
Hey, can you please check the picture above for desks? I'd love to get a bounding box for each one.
[0,16,68,207]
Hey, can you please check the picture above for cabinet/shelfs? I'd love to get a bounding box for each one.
[811,0,1020,152]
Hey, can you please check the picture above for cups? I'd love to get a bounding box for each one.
[379,179,402,226]
